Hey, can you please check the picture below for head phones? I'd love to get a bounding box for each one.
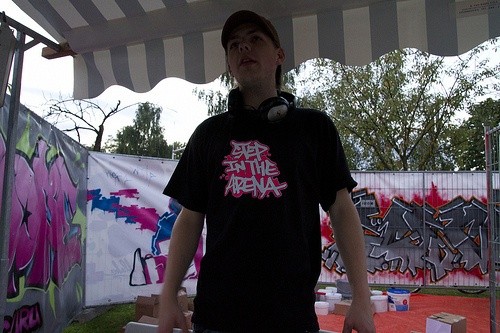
[227,86,296,123]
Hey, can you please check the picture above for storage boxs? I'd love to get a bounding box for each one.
[425,311,467,333]
[334,300,352,316]
[122,286,194,333]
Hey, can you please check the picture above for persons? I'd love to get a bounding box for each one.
[158,9,376,333]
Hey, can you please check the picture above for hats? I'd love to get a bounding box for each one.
[222,9,281,48]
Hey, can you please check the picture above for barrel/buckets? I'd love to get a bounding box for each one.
[371,290,383,295]
[315,287,342,311]
[315,301,329,315]
[388,288,410,311]
[370,295,388,313]
